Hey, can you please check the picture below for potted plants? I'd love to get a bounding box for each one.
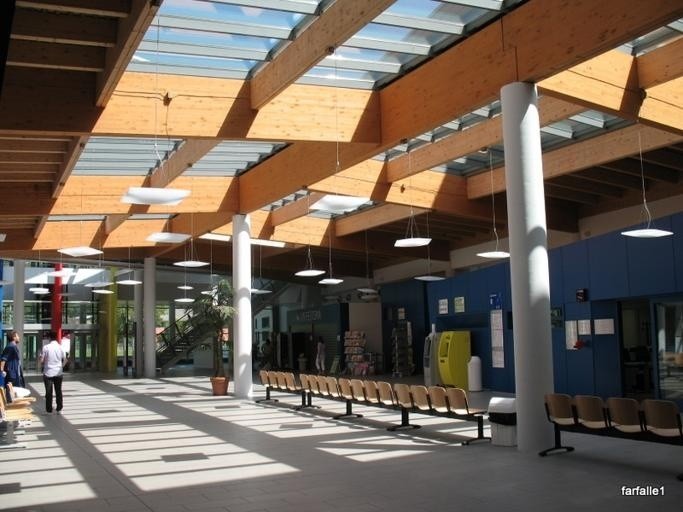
[181,280,235,395]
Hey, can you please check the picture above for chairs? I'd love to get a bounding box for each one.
[538,393,682,482]
[255,369,492,445]
[0,382,37,449]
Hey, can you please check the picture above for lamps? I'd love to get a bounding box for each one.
[28,11,675,304]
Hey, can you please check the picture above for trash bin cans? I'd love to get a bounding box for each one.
[466,356,482,392]
[485,395,517,449]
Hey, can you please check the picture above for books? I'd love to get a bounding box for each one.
[343,329,366,361]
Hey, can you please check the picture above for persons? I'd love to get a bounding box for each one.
[259,339,273,369]
[0,330,26,390]
[313,336,326,376]
[38,329,67,416]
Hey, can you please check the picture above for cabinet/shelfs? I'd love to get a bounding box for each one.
[344,330,366,372]
